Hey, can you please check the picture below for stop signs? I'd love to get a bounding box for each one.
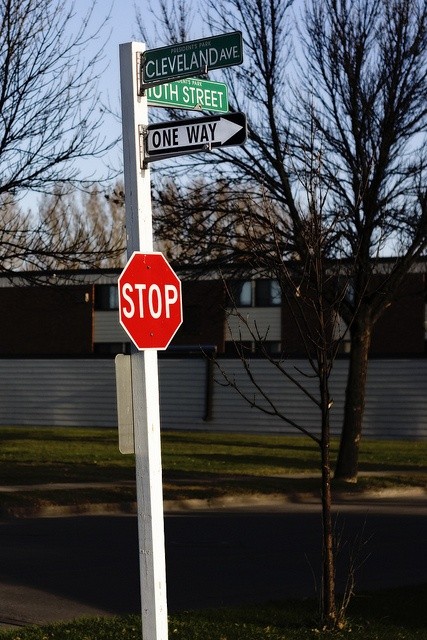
[118,249,182,353]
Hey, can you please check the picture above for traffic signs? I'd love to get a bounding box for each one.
[140,111,248,167]
[140,31,243,94]
[142,77,228,111]
